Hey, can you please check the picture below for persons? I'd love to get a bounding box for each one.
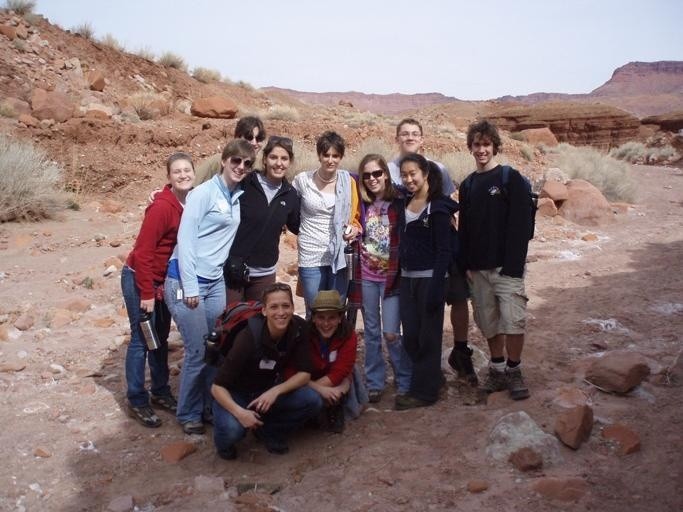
[145,115,265,207]
[159,138,257,435]
[457,119,537,401]
[223,136,299,311]
[353,152,415,405]
[119,152,196,429]
[394,151,458,410]
[289,131,364,324]
[208,281,324,460]
[283,287,369,435]
[386,117,479,388]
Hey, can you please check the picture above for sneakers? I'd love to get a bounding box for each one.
[394,391,433,410]
[213,438,238,461]
[264,437,290,455]
[478,365,507,393]
[183,418,207,436]
[367,389,383,402]
[204,404,218,425]
[127,402,162,428]
[503,365,530,400]
[447,347,477,387]
[149,388,180,415]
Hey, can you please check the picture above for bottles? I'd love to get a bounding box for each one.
[139,308,162,351]
[342,237,354,281]
[530,191,538,216]
[202,331,218,363]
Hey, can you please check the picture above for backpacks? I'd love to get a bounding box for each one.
[465,165,539,242]
[202,299,266,370]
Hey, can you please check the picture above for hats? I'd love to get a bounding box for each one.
[306,289,350,313]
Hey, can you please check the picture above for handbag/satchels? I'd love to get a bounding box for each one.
[223,256,252,291]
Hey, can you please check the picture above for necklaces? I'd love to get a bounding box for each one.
[316,169,337,183]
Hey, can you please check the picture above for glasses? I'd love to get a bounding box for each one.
[243,133,265,142]
[361,169,385,180]
[230,156,255,169]
[267,135,294,148]
